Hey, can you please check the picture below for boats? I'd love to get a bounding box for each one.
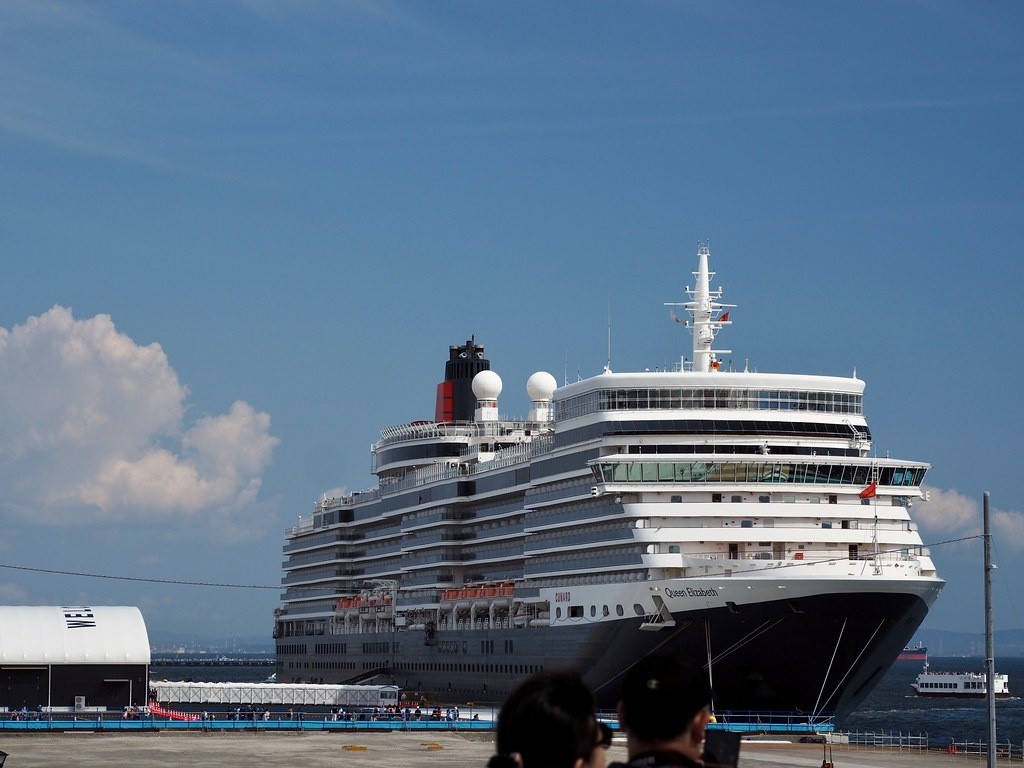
[897,640,928,659]
[909,651,1010,699]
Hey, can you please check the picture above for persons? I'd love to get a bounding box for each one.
[859,481,876,499]
[36,683,480,725]
[485,676,611,768]
[914,665,997,690]
[614,664,717,768]
[719,312,728,323]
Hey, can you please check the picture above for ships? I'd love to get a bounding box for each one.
[271,239,948,735]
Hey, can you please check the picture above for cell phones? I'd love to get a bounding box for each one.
[705,729,743,768]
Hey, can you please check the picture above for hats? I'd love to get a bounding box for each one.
[624,659,710,722]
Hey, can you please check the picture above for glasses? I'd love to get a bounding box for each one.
[592,721,612,751]
